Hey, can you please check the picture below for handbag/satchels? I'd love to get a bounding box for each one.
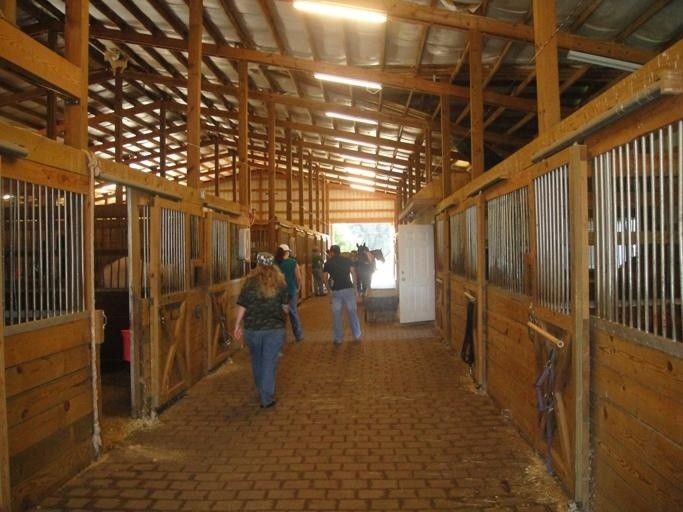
[328,278,334,287]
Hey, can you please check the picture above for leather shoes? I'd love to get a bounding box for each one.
[260,401,276,408]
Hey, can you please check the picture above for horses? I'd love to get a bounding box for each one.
[340,243,385,296]
[100,256,180,290]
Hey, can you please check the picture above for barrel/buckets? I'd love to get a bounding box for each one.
[119,327,130,363]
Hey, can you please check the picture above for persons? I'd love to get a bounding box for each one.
[323,245,361,345]
[312,246,330,296]
[234,251,289,408]
[273,243,304,341]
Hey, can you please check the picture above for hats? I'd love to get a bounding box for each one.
[257,251,274,266]
[279,243,292,252]
